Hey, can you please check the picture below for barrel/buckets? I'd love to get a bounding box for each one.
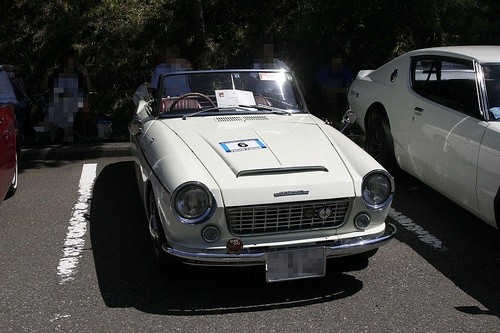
[96,117,113,139]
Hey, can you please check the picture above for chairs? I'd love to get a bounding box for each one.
[423,81,449,97]
[160,96,274,112]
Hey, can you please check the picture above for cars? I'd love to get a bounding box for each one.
[0,65,18,205]
[128,69,397,283]
[347,45,500,227]
